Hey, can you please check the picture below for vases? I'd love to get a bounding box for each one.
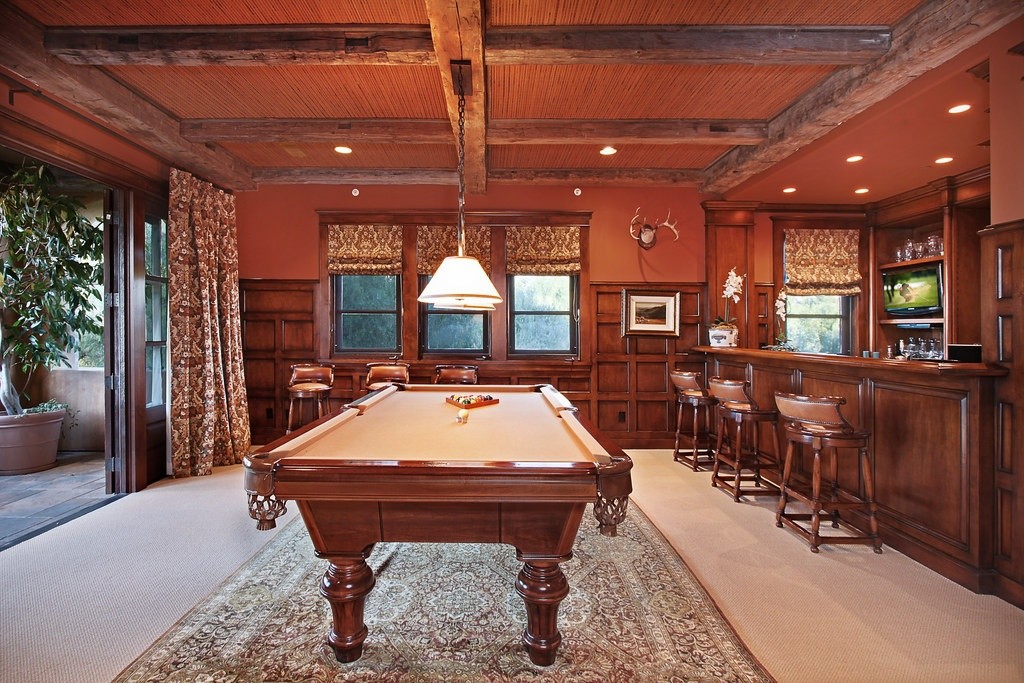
[775,336,795,352]
[706,316,740,347]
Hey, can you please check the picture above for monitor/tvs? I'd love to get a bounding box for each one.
[880,263,943,316]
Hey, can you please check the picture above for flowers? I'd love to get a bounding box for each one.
[721,266,746,323]
[775,286,788,341]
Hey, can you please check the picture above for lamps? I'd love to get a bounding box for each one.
[415,66,504,311]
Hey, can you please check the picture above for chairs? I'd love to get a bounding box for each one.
[434,364,480,385]
[773,387,884,555]
[709,374,789,504]
[285,362,337,434]
[667,368,736,472]
[363,360,412,393]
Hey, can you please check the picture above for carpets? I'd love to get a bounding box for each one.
[104,495,782,683]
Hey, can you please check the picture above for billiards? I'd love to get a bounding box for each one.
[459,408,469,418]
[450,394,493,404]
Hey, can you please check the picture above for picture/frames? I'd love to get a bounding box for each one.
[620,286,681,339]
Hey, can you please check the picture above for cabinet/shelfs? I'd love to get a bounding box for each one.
[871,213,946,361]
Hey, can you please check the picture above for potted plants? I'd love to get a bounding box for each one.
[1,148,103,478]
